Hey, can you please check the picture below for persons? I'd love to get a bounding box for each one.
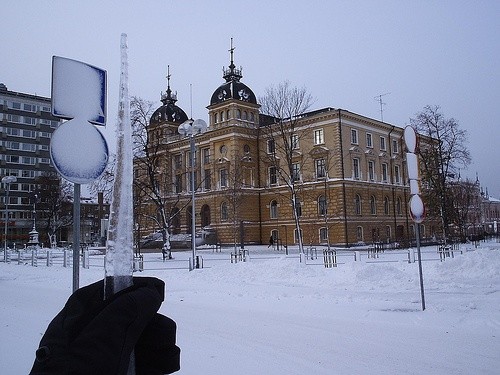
[29,276,180,375]
[268,236,275,248]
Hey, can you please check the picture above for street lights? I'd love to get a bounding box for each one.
[178,117,208,269]
[1,175,18,264]
